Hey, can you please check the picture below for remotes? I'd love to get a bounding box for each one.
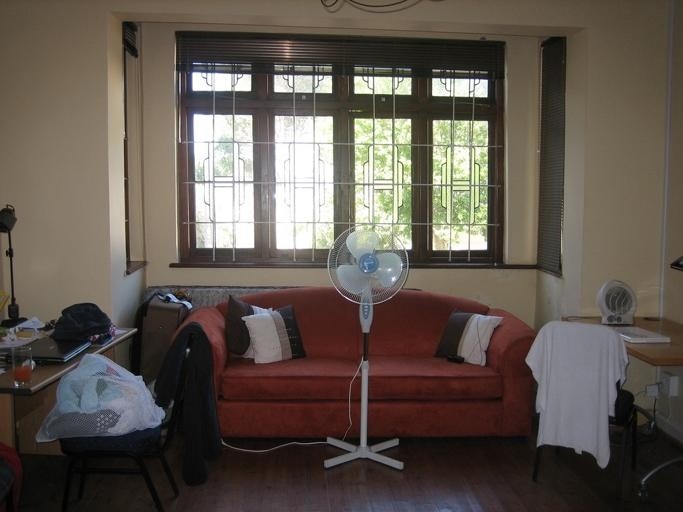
[448,353,464,364]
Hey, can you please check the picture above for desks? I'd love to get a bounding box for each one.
[0,326,137,407]
[561,315,682,499]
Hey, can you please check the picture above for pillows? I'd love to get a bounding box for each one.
[433,308,504,367]
[225,293,305,364]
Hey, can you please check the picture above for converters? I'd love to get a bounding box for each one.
[646,385,659,397]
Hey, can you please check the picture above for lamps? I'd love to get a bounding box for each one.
[1,206,27,327]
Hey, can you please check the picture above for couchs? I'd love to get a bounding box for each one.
[168,285,538,438]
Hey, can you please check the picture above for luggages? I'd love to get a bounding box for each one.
[137,291,193,383]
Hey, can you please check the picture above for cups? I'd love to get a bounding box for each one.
[11,346,33,387]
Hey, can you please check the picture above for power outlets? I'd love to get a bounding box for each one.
[659,373,679,398]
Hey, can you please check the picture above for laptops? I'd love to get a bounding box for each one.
[5,338,92,363]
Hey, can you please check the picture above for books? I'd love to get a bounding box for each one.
[0,320,54,368]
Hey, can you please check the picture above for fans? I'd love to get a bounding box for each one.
[325,223,407,472]
[597,279,638,324]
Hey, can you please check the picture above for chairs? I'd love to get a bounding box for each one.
[52,324,216,511]
[532,321,638,498]
